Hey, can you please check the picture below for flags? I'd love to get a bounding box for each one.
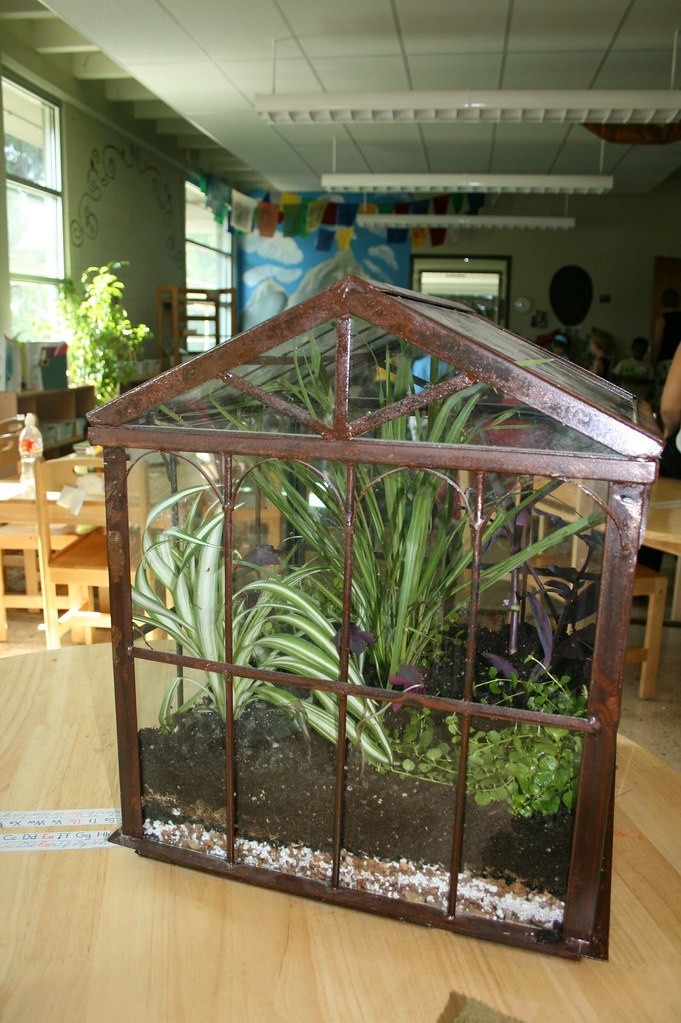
[192,170,485,253]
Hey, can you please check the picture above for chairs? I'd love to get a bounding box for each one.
[508,467,668,701]
[0,412,168,653]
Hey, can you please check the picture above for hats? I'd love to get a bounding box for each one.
[554,334,567,344]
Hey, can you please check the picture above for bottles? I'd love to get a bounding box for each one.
[18,413,43,492]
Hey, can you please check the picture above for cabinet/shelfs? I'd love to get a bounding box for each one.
[0,381,96,481]
[153,283,238,370]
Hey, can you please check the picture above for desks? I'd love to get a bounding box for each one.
[0,445,246,647]
[514,469,680,625]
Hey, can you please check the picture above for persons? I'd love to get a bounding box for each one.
[535,319,681,607]
[613,337,654,402]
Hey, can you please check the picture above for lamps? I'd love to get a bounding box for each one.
[250,28,681,235]
